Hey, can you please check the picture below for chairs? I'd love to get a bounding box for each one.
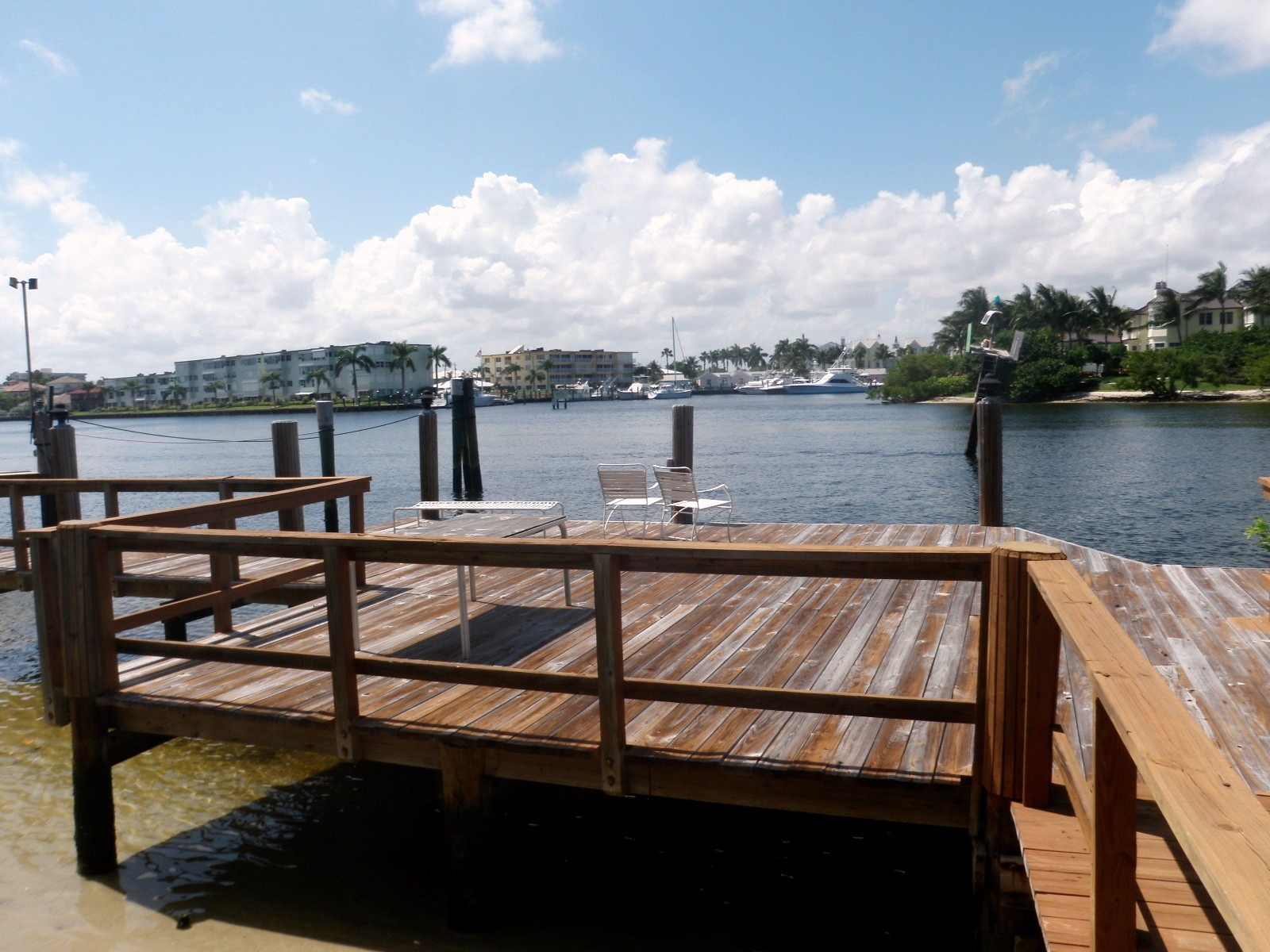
[597,463,732,543]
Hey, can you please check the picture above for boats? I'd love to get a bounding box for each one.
[783,340,870,395]
[733,363,810,395]
[447,388,515,407]
[591,374,659,400]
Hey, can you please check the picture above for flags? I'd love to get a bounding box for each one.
[474,350,481,358]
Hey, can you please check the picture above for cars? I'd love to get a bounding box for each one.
[681,382,700,389]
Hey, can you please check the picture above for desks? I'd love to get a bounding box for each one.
[365,513,572,659]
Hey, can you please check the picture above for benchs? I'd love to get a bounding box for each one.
[392,501,564,533]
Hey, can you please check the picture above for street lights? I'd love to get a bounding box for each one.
[9,277,38,433]
[647,368,651,376]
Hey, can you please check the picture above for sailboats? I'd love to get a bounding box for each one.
[648,317,694,399]
